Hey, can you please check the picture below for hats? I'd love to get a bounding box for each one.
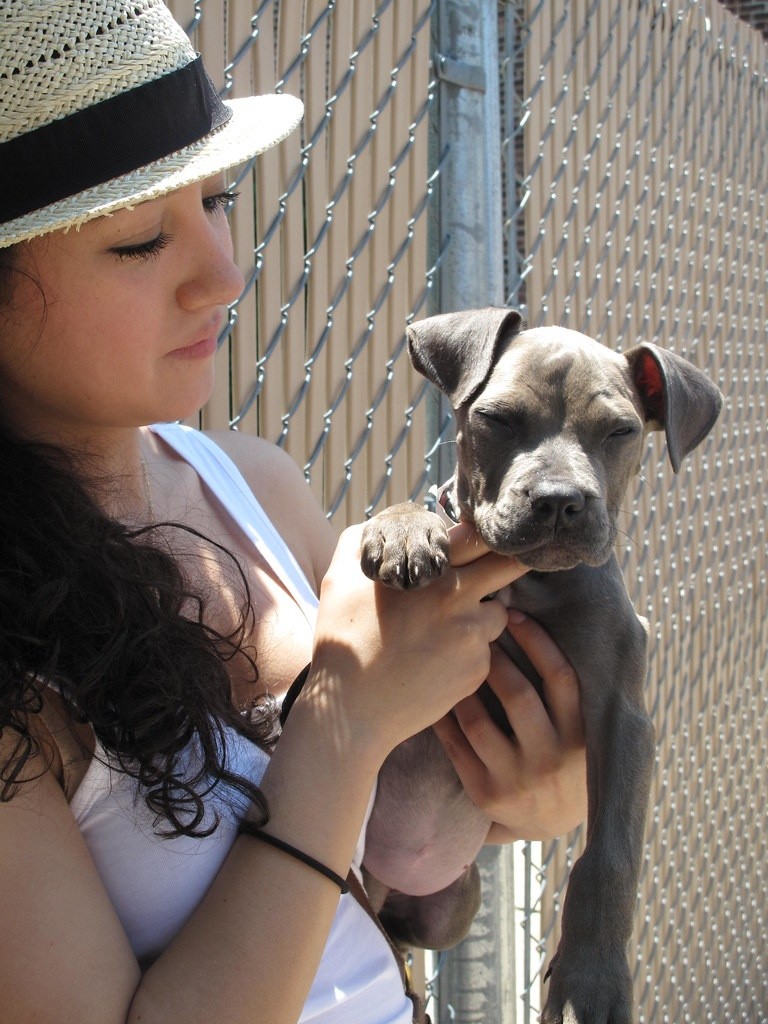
[0,0,304,248]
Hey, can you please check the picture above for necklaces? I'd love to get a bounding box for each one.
[141,457,160,608]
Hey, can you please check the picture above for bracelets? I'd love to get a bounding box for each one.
[239,822,349,894]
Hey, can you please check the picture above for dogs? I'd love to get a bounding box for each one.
[279,306,724,1024]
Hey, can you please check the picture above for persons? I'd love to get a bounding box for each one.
[0,0,589,1024]
